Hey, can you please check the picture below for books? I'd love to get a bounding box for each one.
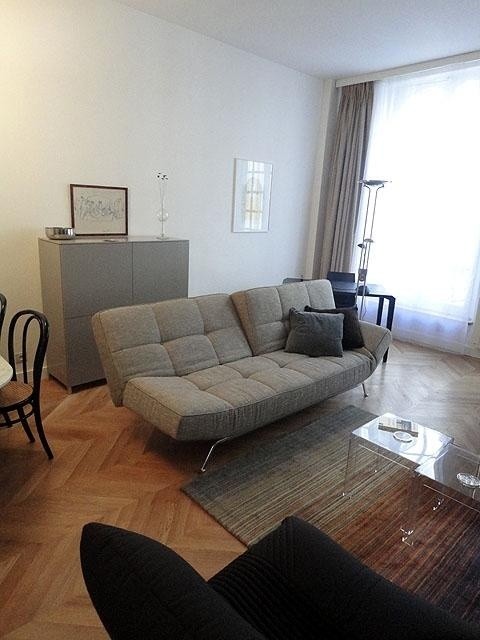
[378,415,419,437]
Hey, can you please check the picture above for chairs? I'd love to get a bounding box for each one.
[0,294,54,460]
[326,270,357,318]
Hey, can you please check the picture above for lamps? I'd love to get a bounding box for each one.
[357,178,391,318]
[151,167,170,240]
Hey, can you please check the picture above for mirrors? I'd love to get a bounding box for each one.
[232,158,273,232]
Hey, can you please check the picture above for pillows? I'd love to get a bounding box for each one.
[284,303,364,357]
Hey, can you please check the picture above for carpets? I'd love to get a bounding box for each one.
[179,401,480,640]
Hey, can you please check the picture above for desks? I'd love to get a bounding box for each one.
[282,276,396,362]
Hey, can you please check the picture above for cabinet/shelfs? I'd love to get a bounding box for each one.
[36,238,189,393]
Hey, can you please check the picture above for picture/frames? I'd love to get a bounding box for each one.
[70,184,130,236]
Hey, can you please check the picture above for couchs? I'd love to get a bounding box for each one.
[89,279,393,472]
[78,514,478,640]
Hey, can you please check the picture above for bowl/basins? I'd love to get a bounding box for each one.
[45,227,74,240]
